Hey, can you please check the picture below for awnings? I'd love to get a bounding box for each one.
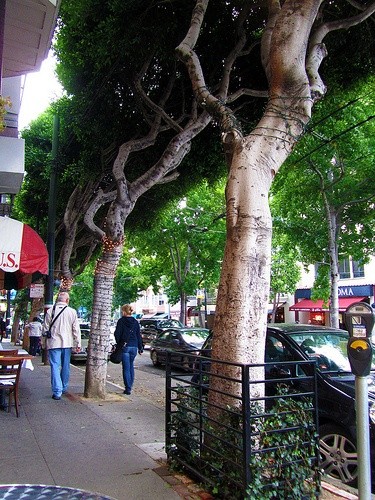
[0,216,49,290]
[288,296,367,311]
[191,302,286,316]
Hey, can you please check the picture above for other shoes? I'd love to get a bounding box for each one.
[52,394,61,400]
[124,390,131,395]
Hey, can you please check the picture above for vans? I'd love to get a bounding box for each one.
[191,322,375,488]
[139,318,186,348]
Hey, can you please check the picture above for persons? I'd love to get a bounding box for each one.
[114,304,143,395]
[42,292,82,400]
[0,317,10,342]
[25,312,43,356]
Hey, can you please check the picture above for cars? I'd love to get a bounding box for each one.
[149,327,212,371]
[70,329,116,364]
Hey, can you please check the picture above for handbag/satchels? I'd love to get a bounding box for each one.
[46,331,51,338]
[111,343,123,364]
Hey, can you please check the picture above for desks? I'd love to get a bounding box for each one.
[0,354,37,407]
[0,349,35,371]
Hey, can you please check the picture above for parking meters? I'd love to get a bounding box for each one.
[344,301,375,500]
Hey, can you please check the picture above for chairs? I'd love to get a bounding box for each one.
[0,354,25,418]
[0,350,19,369]
